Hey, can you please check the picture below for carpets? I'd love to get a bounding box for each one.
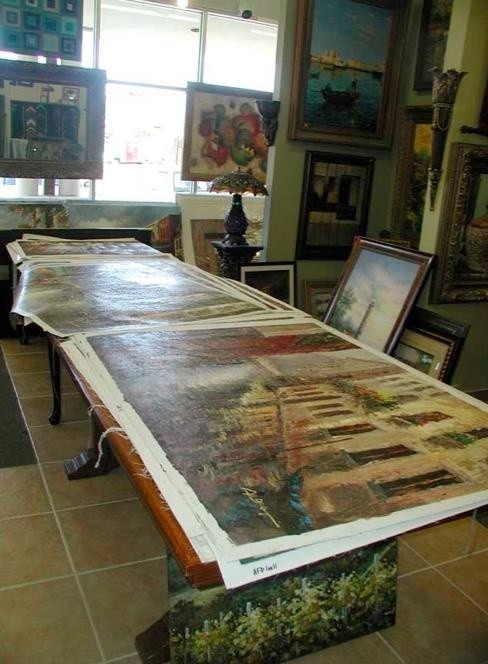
[0,347,39,471]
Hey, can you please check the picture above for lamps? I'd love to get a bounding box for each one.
[209,166,270,246]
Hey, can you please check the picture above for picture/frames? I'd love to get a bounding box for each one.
[181,78,275,184]
[0,58,107,180]
